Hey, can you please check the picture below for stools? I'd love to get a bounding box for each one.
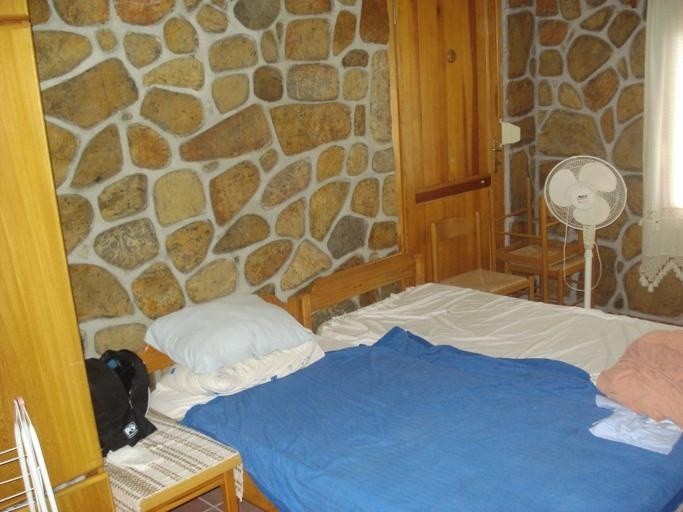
[504,255,585,306]
[105,412,241,512]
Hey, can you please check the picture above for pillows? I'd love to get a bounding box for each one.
[156,334,325,393]
[145,292,314,372]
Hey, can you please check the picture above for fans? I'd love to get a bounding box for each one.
[543,154,628,309]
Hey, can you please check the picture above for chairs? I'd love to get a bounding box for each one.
[489,177,585,301]
[429,212,535,302]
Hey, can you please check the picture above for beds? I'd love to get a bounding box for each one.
[140,253,683,512]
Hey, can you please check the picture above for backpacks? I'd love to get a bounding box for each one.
[84,346,156,458]
